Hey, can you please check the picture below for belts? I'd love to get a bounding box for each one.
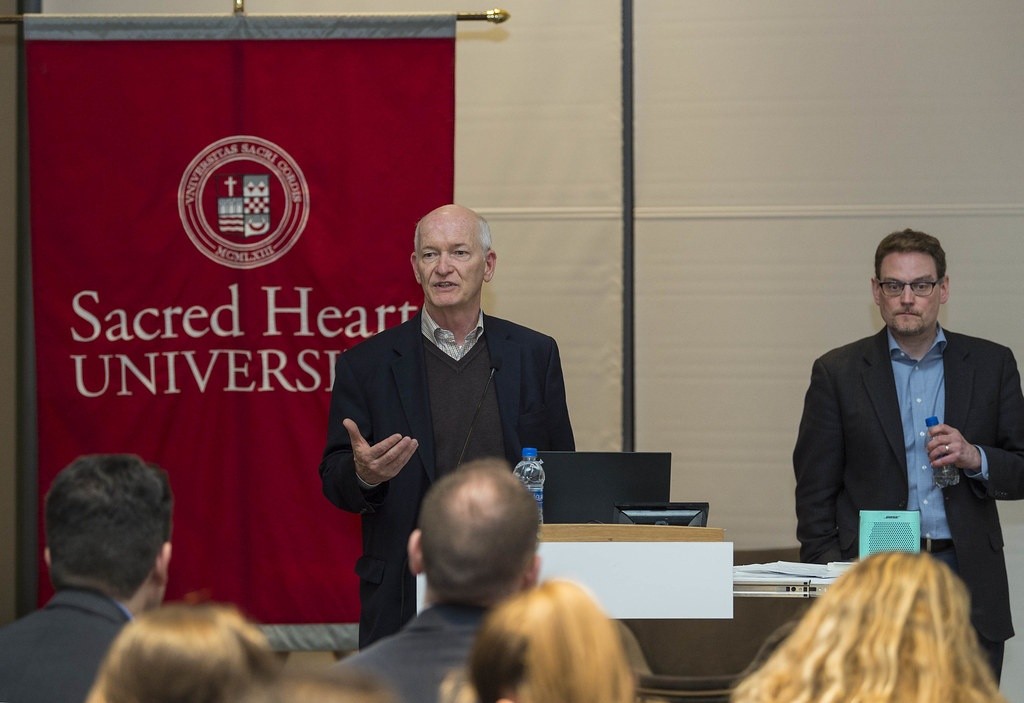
[920,537,953,554]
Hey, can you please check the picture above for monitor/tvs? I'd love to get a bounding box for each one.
[613,503,709,527]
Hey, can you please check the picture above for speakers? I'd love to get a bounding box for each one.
[858,510,920,562]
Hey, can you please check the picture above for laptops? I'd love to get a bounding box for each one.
[536,451,671,525]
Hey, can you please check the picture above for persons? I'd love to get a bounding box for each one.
[792,227,1023,695]
[319,204,576,653]
[727,550,1013,703]
[0,453,172,703]
[437,581,638,703]
[315,458,540,703]
[86,601,393,703]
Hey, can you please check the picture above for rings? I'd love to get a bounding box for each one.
[945,445,950,454]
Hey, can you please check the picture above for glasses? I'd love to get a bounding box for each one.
[876,278,941,297]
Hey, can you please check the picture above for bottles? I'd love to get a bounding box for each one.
[925,414,962,488]
[510,447,546,525]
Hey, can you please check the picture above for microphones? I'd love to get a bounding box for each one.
[457,354,503,467]
